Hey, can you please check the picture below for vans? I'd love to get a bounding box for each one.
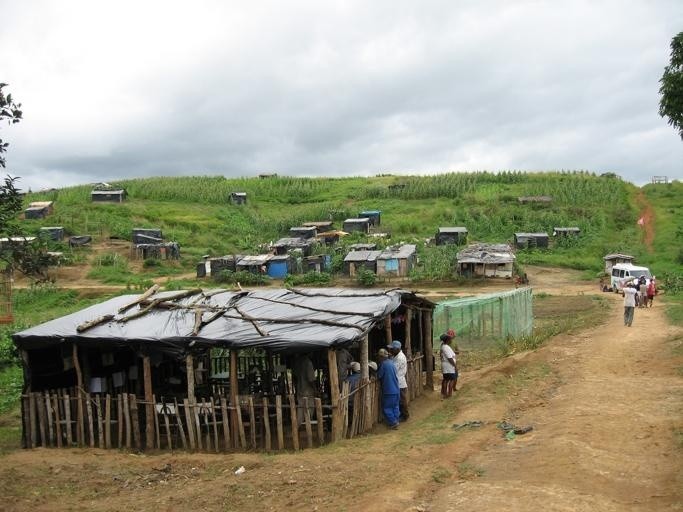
[611,263,657,293]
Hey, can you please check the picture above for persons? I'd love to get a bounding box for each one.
[318,341,410,430]
[440,329,458,397]
[622,275,656,327]
[293,350,317,429]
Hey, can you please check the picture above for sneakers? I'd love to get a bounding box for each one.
[441,388,458,399]
[390,422,400,430]
[399,414,408,422]
[624,321,631,327]
[636,304,653,308]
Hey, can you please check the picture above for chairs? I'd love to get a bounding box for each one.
[154,402,260,448]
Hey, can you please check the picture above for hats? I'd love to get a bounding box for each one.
[350,361,361,373]
[439,330,454,342]
[368,361,378,371]
[385,340,402,349]
[378,347,389,358]
